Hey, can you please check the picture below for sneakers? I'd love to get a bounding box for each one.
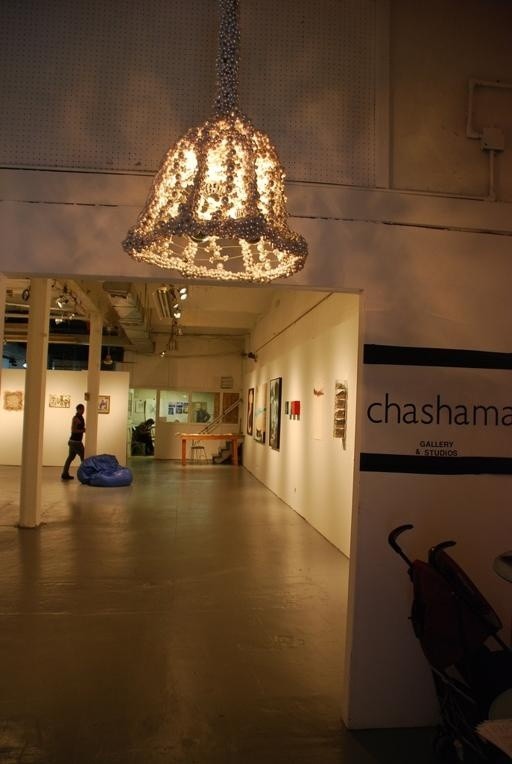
[62,474,75,479]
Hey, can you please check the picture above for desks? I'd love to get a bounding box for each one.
[178,433,246,465]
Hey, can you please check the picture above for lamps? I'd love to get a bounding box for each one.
[103,347,113,365]
[122,0,308,284]
[55,295,73,324]
[174,287,188,337]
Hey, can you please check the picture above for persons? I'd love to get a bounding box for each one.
[190,401,211,423]
[60,402,86,480]
[132,417,155,457]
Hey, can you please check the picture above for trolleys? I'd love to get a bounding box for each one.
[387,520,510,764]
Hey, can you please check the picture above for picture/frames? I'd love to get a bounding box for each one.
[3,391,22,411]
[98,395,110,414]
[268,377,281,449]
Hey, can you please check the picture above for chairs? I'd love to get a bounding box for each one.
[132,425,146,455]
[190,446,208,465]
